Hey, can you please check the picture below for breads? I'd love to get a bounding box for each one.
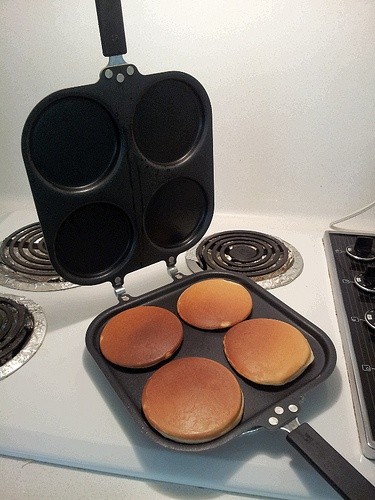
[176,279,253,330]
[99,305,182,370]
[223,318,315,387]
[141,358,246,444]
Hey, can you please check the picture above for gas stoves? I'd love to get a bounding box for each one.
[1,209,375,500]
